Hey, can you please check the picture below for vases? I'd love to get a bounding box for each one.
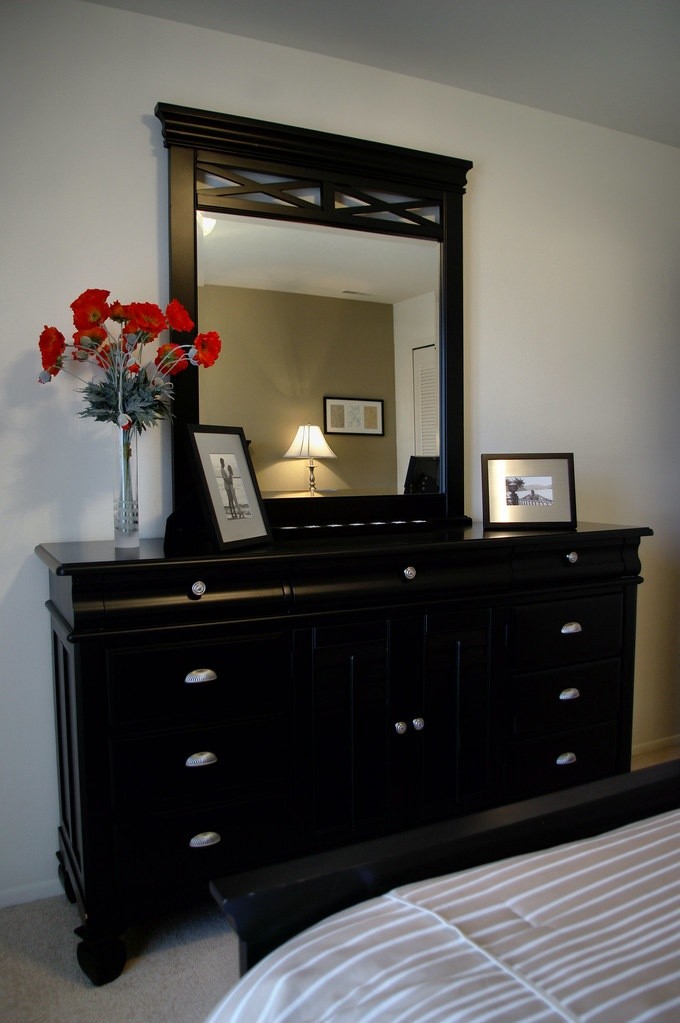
[112,421,140,547]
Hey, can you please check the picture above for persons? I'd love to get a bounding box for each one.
[220,458,244,518]
[529,490,538,500]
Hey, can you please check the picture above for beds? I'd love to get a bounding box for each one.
[205,752,679,1023]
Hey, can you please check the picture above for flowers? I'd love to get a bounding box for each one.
[38,289,218,474]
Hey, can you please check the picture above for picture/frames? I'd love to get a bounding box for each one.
[482,453,578,531]
[320,394,386,439]
[188,425,275,550]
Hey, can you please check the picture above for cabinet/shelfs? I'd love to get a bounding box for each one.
[34,524,656,987]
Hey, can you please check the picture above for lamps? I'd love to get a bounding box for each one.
[284,423,337,495]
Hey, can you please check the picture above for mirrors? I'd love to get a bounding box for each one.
[197,212,442,502]
